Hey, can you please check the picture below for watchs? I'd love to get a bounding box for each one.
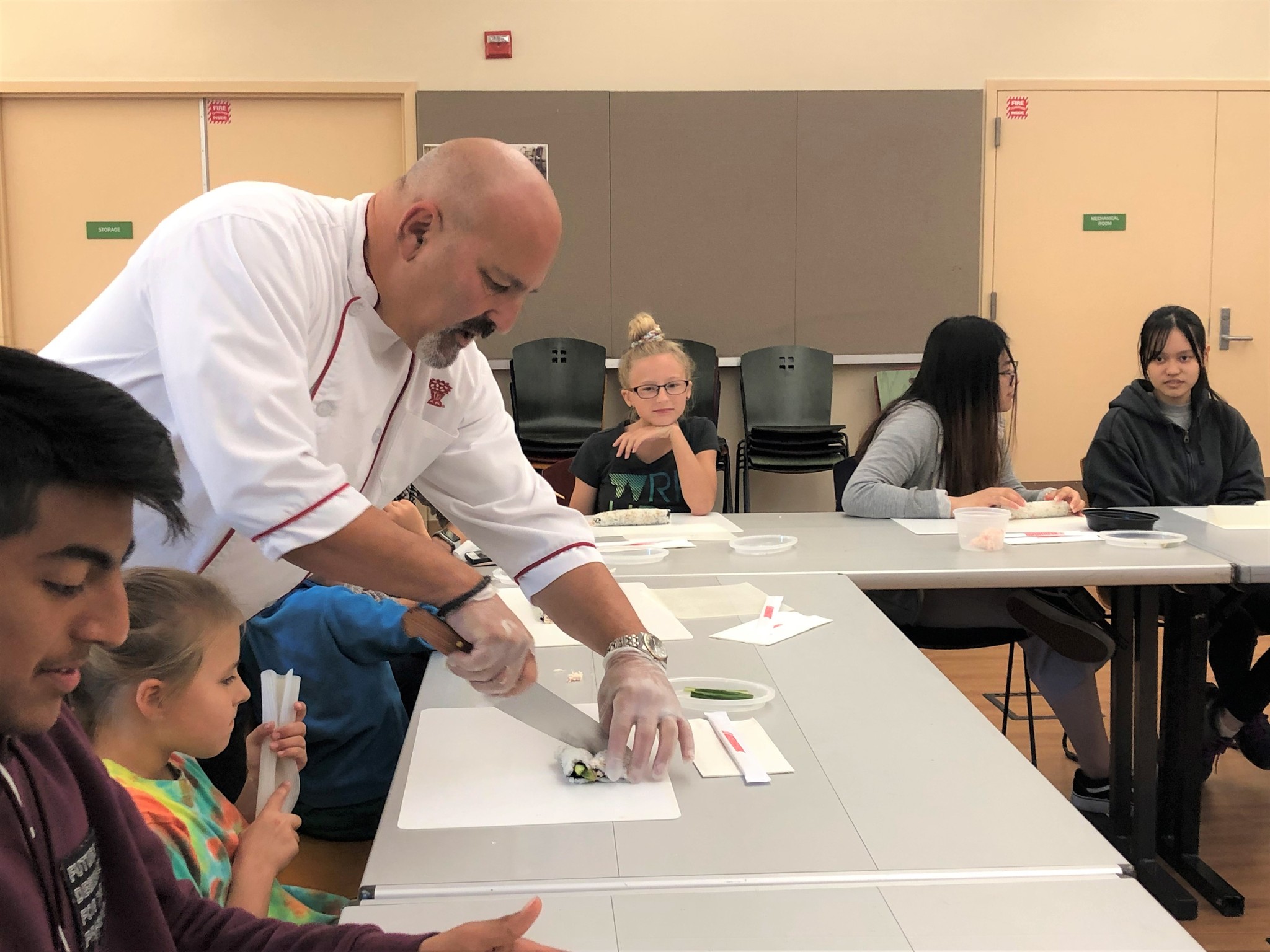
[432,528,461,549]
[606,632,668,669]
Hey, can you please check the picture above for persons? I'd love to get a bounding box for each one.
[567,310,721,516]
[59,564,336,933]
[392,483,469,553]
[841,315,1116,819]
[33,137,695,783]
[237,498,444,845]
[0,345,563,952]
[1081,305,1270,781]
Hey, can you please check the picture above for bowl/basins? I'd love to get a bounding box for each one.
[953,506,1011,552]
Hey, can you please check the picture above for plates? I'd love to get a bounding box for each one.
[595,546,669,564]
[667,678,775,711]
[1098,529,1186,547]
[729,535,797,554]
[1082,509,1160,533]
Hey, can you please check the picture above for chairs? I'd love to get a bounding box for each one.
[1062,456,1269,763]
[665,338,849,513]
[875,368,920,412]
[508,337,607,507]
[832,455,1038,768]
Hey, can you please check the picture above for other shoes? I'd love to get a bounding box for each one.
[1200,682,1235,782]
[1233,711,1270,770]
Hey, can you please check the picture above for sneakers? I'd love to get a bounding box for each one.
[1070,767,1134,817]
[1006,588,1115,663]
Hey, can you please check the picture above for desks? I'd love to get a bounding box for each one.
[450,503,1235,922]
[335,573,1210,952]
[1106,499,1270,917]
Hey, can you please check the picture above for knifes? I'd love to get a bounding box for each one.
[399,606,640,771]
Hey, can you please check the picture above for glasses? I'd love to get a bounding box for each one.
[998,361,1018,386]
[623,379,691,399]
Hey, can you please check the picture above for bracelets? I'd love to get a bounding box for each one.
[438,575,490,617]
